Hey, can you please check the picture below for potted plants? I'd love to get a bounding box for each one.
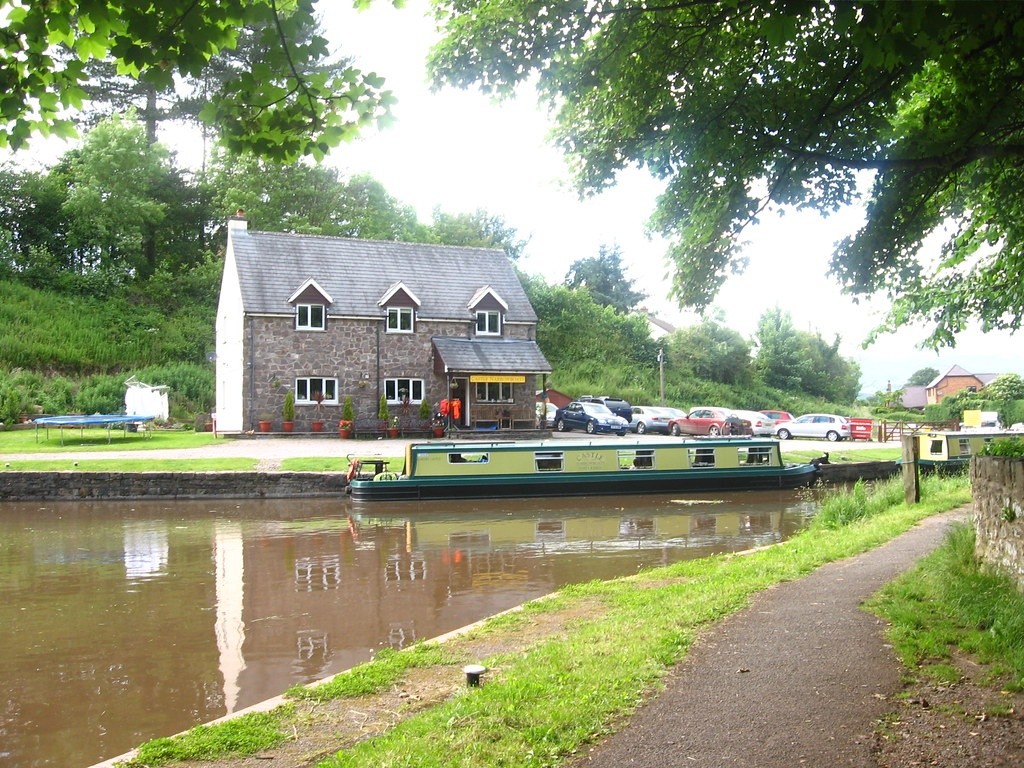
[311,391,324,432]
[258,413,272,432]
[281,389,295,432]
[205,417,212,432]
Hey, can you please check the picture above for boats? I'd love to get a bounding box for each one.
[343,435,824,501]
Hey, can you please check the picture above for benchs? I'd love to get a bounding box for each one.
[510,406,536,429]
[471,404,500,430]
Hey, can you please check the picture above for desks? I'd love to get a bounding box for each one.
[500,417,511,430]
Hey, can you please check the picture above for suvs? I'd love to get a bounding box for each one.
[574,394,633,424]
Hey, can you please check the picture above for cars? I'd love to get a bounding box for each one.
[555,401,630,436]
[667,408,752,439]
[629,405,676,434]
[731,410,776,438]
[757,410,794,437]
[772,413,852,442]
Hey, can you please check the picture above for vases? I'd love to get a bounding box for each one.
[340,429,351,439]
[433,428,444,438]
[20,418,26,423]
[388,428,398,439]
[127,424,138,432]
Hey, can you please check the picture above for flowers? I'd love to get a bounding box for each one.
[431,413,444,428]
[389,416,399,428]
[339,419,352,429]
